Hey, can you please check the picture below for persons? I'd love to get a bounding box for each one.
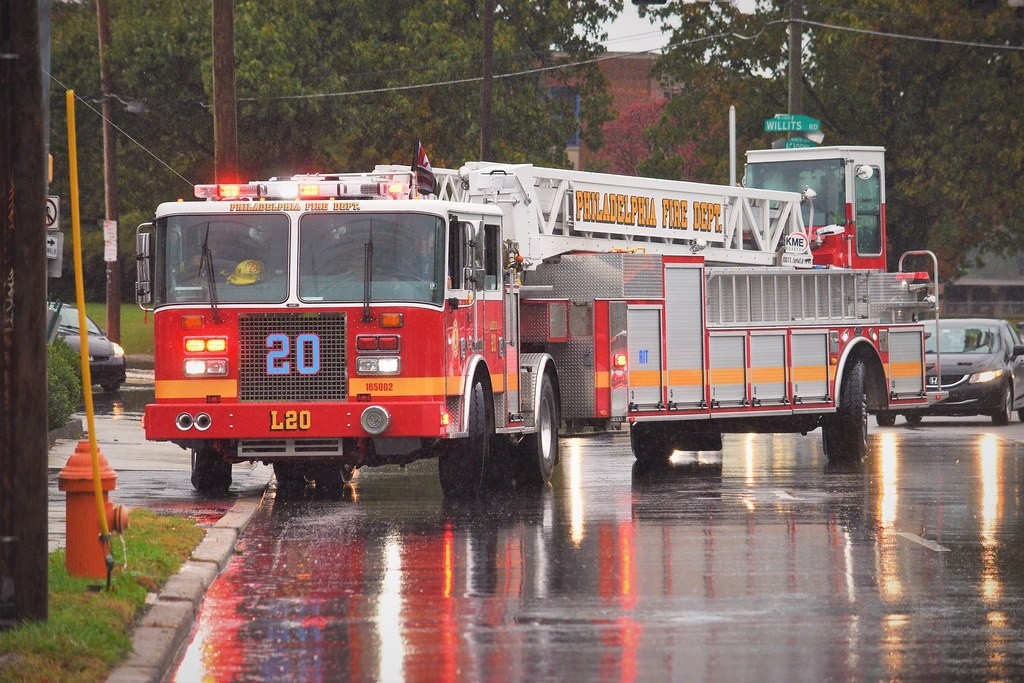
[373,222,453,289]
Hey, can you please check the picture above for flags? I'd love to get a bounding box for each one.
[410,142,437,196]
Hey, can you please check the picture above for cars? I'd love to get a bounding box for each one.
[49,301,127,391]
[878,318,1024,425]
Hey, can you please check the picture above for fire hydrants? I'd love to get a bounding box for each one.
[58,440,129,577]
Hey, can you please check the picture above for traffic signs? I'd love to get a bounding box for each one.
[764,113,820,131]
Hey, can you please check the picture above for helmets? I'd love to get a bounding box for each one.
[227,260,266,285]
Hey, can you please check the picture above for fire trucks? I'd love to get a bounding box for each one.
[130,143,948,500]
